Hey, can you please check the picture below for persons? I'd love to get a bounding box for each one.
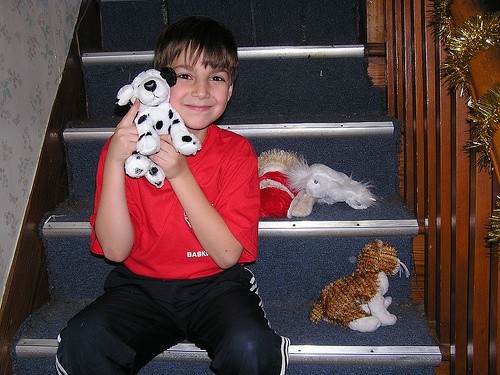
[54,18,291,375]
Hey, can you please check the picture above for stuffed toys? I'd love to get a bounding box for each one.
[256,150,410,332]
[114,66,201,188]
